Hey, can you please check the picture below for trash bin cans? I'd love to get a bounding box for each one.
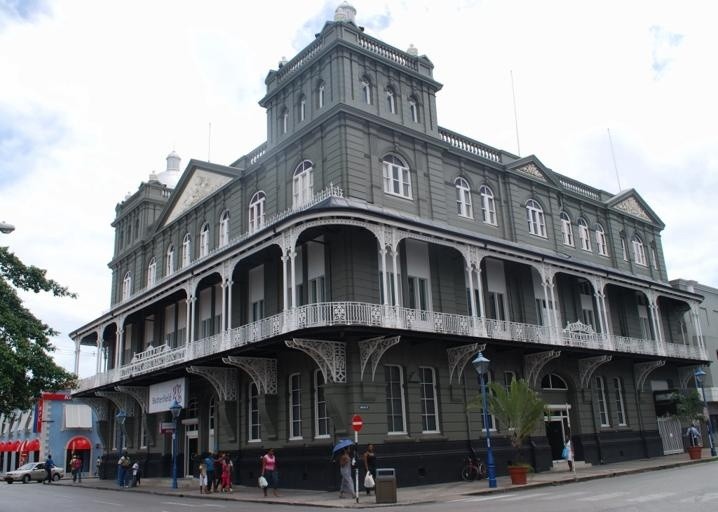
[375,468,397,503]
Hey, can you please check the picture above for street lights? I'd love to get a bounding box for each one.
[166,397,185,490]
[691,365,716,457]
[470,349,500,489]
[114,408,127,459]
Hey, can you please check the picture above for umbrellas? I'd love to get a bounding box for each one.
[331,439,355,456]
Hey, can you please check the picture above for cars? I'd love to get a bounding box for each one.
[3,460,66,486]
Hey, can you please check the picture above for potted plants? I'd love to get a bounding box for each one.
[465,378,550,485]
[670,389,708,460]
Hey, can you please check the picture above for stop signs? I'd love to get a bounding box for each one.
[350,415,365,433]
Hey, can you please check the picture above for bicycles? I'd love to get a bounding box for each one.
[458,455,487,483]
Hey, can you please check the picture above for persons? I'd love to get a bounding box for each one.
[563,436,575,472]
[338,446,356,499]
[45,454,56,483]
[685,423,702,446]
[261,447,280,498]
[132,459,140,480]
[363,443,376,496]
[198,452,234,494]
[96,455,101,477]
[117,451,130,489]
[70,455,82,483]
